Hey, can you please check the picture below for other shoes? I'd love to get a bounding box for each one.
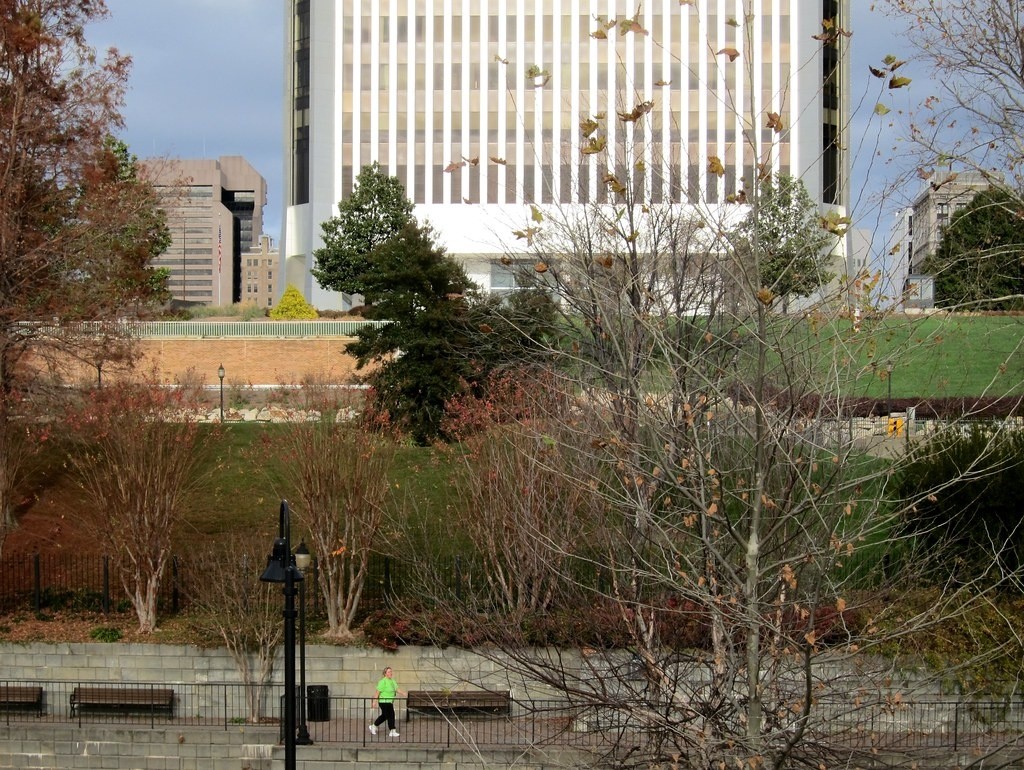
[389,732,400,736]
[368,725,376,735]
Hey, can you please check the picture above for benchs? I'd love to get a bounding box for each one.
[406,691,511,723]
[0,687,43,718]
[70,688,174,720]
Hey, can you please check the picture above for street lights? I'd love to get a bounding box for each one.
[886,360,893,418]
[94,347,102,387]
[218,362,225,422]
[260,498,305,770]
[295,536,312,745]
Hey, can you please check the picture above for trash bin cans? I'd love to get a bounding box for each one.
[307,685,329,722]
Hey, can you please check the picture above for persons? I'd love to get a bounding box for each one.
[369,667,408,737]
